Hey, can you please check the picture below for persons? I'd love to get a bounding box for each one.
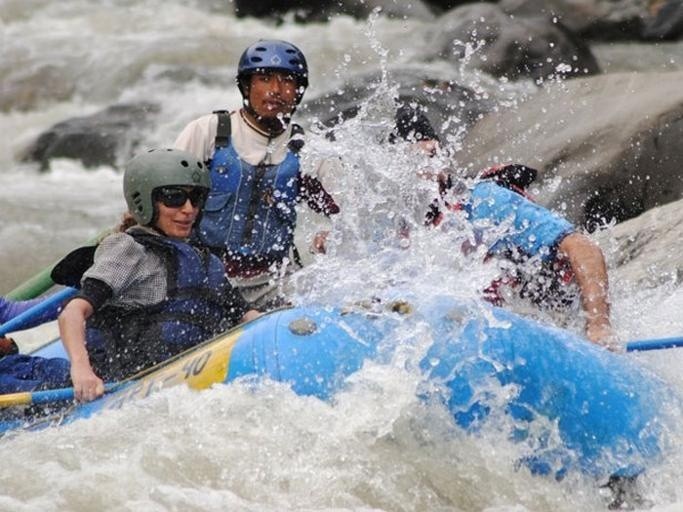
[309,105,622,353]
[0,294,75,393]
[114,38,339,316]
[59,147,264,405]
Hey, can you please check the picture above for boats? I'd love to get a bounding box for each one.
[0,289,682,487]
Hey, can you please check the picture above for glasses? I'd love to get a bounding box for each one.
[153,185,208,209]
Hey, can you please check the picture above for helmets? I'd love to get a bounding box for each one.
[237,39,308,105]
[124,146,213,227]
